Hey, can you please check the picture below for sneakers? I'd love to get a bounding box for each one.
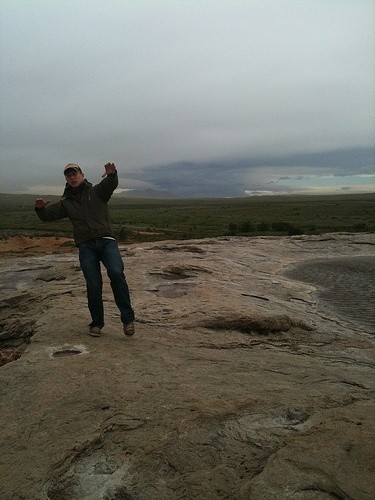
[123,321,135,336]
[90,325,102,337]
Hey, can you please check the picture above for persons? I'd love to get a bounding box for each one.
[35,162,135,337]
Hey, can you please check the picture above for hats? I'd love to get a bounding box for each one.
[63,164,81,174]
